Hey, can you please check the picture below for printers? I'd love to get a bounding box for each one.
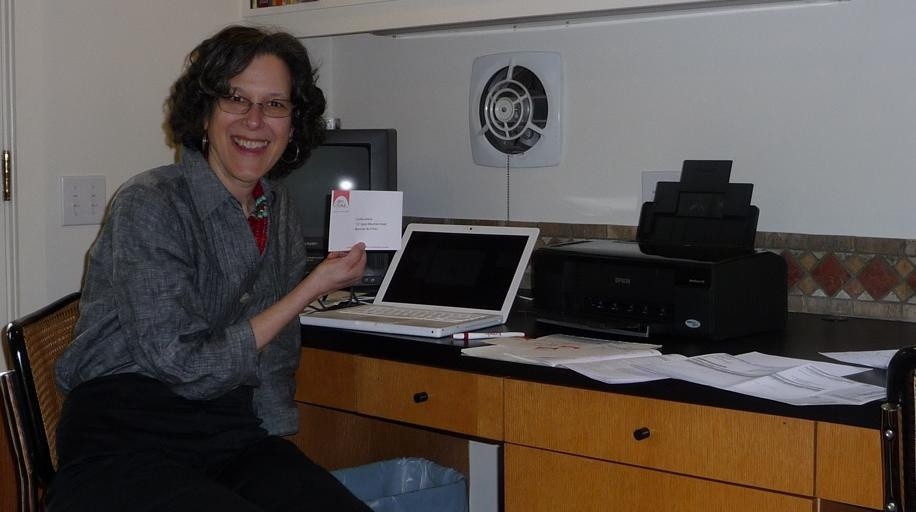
[528,160,789,355]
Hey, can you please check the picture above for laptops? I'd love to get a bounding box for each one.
[299,223,540,337]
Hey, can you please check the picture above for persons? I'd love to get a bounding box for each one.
[40,25,374,512]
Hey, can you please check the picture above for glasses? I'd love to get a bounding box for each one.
[214,93,299,119]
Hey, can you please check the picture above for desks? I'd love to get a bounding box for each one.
[295,313,916,512]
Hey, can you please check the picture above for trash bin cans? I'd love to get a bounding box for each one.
[328,457,468,512]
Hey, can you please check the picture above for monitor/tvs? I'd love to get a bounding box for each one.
[276,128,397,287]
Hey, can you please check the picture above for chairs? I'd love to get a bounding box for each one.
[1,291,80,479]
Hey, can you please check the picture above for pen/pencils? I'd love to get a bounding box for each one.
[452,332,525,339]
